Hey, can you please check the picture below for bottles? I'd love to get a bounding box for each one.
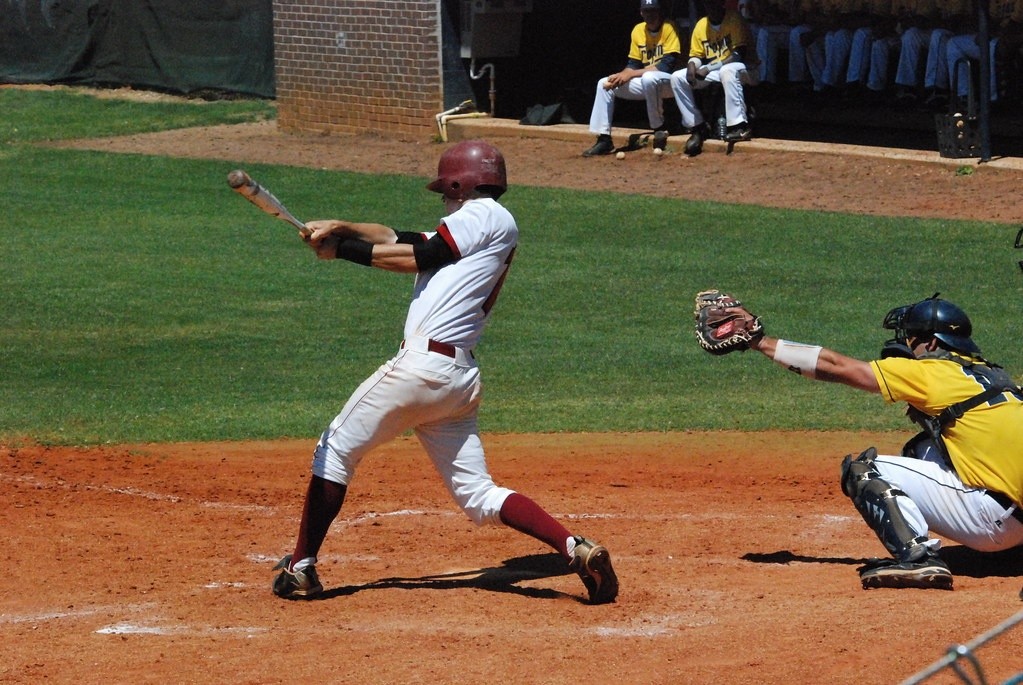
[718,115,726,140]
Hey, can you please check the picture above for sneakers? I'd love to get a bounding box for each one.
[722,127,751,142]
[685,121,710,151]
[583,136,614,157]
[860,553,954,591]
[569,536,619,606]
[271,555,325,597]
[653,123,668,139]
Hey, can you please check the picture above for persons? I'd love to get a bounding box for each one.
[727,0,1023,103]
[670,0,761,152]
[696,288,1023,589]
[268,142,618,605]
[582,0,690,157]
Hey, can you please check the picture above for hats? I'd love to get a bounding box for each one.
[640,1,660,10]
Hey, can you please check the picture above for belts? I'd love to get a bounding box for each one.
[986,490,1023,524]
[402,338,476,359]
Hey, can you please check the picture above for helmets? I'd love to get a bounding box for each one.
[424,142,508,201]
[906,296,982,354]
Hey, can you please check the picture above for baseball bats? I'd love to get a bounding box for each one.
[227,169,314,238]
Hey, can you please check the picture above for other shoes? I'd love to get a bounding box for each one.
[751,77,972,112]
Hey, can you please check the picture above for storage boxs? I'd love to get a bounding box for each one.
[934,115,982,158]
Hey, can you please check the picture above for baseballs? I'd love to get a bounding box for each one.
[615,151,625,159]
[654,147,662,156]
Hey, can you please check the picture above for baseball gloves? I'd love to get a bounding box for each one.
[694,288,765,356]
